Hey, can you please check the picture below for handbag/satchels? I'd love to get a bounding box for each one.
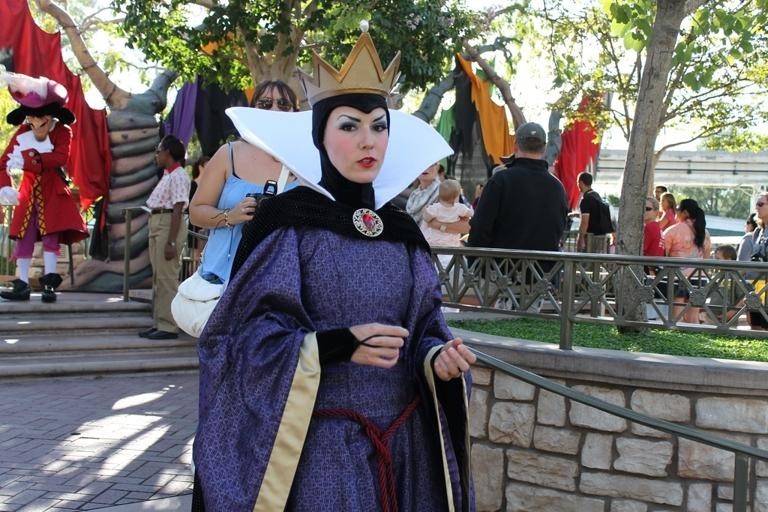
[171,272,224,338]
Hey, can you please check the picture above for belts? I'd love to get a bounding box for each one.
[151,209,180,214]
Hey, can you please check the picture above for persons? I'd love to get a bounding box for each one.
[577,171,618,272]
[137,135,192,340]
[0,73,90,303]
[706,245,745,326]
[406,163,474,295]
[186,80,300,284]
[464,121,567,315]
[644,186,711,324]
[738,193,768,330]
[189,28,482,512]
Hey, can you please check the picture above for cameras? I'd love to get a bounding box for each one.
[752,247,767,262]
[246,180,278,215]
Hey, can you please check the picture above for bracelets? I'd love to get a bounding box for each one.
[222,211,233,229]
[168,240,177,247]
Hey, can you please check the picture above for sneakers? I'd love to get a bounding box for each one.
[138,328,178,338]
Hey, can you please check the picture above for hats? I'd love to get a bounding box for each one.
[296,20,403,108]
[515,122,545,140]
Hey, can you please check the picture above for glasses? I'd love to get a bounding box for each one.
[255,97,295,111]
